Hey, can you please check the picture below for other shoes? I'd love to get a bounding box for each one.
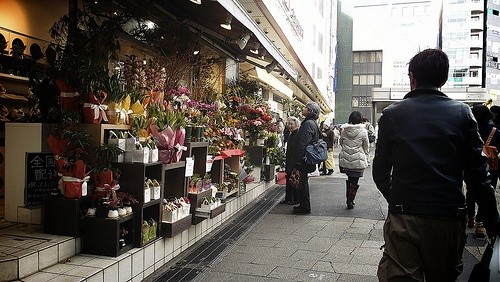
[290,201,298,205]
[475,227,486,238]
[280,200,291,203]
[293,206,311,214]
[468,218,474,228]
[328,170,334,175]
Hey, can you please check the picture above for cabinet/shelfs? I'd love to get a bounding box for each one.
[42,123,276,259]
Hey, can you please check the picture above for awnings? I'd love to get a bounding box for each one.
[155,0,298,82]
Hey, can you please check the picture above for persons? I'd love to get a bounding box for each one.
[372,48,500,282]
[466,104,500,239]
[275,116,300,204]
[292,102,320,214]
[364,118,379,166]
[338,111,370,209]
[318,120,343,175]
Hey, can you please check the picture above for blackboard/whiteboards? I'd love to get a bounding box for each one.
[23,152,64,209]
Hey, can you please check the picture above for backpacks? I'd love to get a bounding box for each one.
[480,128,499,171]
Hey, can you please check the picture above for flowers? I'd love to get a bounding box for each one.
[74,56,278,161]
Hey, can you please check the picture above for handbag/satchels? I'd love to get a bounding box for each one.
[305,141,327,165]
[289,168,303,188]
[369,136,375,143]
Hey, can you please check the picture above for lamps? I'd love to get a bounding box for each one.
[279,73,284,77]
[250,42,259,54]
[285,78,290,82]
[235,33,250,50]
[220,14,233,30]
[256,49,266,60]
[265,62,276,74]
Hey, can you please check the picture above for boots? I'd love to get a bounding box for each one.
[345,181,359,209]
